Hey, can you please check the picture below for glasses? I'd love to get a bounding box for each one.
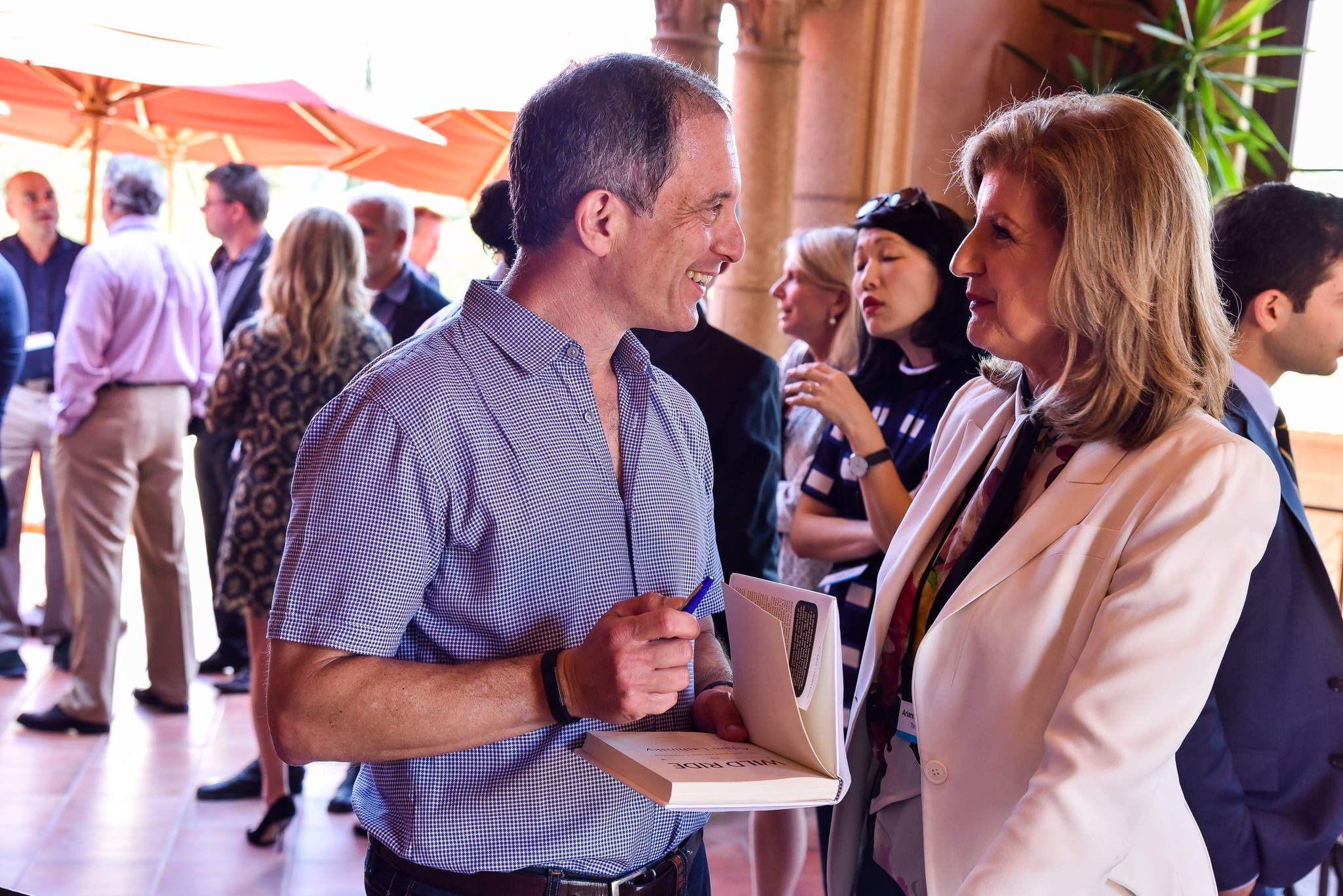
[856,189,941,223]
[204,196,228,208]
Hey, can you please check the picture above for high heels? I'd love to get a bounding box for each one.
[246,797,296,854]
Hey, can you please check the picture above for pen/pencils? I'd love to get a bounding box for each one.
[678,574,715,615]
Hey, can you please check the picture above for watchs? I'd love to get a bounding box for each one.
[848,446,892,477]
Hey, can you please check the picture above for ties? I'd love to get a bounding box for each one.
[1271,406,1302,502]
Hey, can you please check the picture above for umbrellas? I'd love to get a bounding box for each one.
[328,106,522,202]
[0,47,449,248]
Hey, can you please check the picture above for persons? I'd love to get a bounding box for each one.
[405,205,447,295]
[624,182,1343,896]
[409,180,519,334]
[14,151,224,736]
[265,52,748,896]
[826,92,1282,896]
[198,161,274,693]
[0,253,31,551]
[0,171,73,677]
[196,191,451,815]
[210,206,392,854]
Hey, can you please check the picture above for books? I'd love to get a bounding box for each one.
[573,573,852,811]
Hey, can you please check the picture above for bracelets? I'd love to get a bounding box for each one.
[540,647,581,724]
[699,680,733,694]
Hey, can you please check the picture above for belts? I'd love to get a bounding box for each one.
[364,826,710,896]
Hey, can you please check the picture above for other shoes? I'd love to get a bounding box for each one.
[132,686,189,712]
[329,766,361,815]
[196,755,306,801]
[51,639,75,672]
[215,666,253,693]
[198,645,229,673]
[17,704,111,735]
[1,650,27,675]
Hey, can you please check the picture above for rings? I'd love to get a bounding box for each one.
[808,383,815,394]
